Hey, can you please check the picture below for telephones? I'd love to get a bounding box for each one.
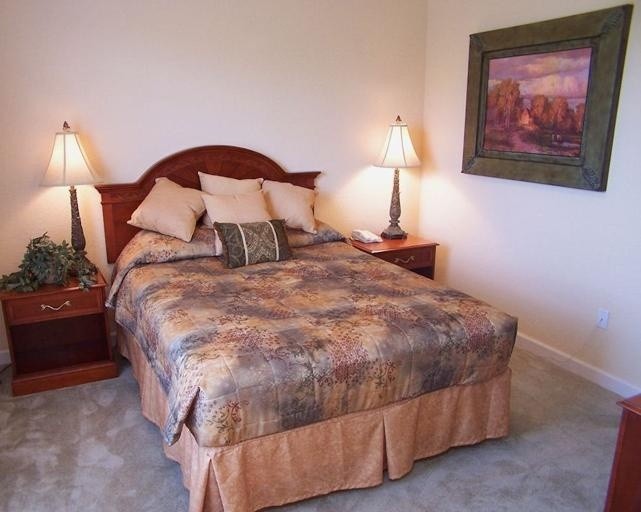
[351,229,383,244]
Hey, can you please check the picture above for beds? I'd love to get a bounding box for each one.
[92,144,521,512]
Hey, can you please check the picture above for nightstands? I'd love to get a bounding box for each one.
[348,233,441,281]
[0,267,120,398]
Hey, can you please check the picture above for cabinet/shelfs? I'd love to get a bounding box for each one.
[600,387,641,512]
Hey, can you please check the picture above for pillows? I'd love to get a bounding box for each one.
[262,178,318,236]
[212,218,292,270]
[197,170,264,227]
[126,177,208,242]
[200,190,273,256]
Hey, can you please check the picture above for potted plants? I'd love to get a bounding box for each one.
[0,229,98,295]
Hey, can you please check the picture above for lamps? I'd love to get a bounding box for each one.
[369,114,423,238]
[39,118,105,277]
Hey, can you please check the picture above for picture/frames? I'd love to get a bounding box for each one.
[461,2,635,192]
